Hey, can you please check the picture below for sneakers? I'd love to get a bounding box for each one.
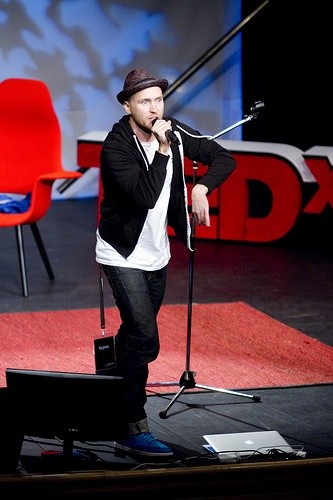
[111,431,177,458]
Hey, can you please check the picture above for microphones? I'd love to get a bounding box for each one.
[152,117,181,146]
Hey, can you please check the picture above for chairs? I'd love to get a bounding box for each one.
[0,78,83,298]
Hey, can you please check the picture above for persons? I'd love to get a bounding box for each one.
[92,66,238,459]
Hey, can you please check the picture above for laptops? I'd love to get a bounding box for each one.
[202,431,294,463]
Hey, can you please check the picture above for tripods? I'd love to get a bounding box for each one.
[146,160,261,418]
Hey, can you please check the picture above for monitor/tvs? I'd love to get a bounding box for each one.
[4,368,130,470]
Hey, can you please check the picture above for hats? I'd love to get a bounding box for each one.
[116,67,171,104]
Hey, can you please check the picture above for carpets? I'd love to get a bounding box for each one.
[0,302,333,397]
